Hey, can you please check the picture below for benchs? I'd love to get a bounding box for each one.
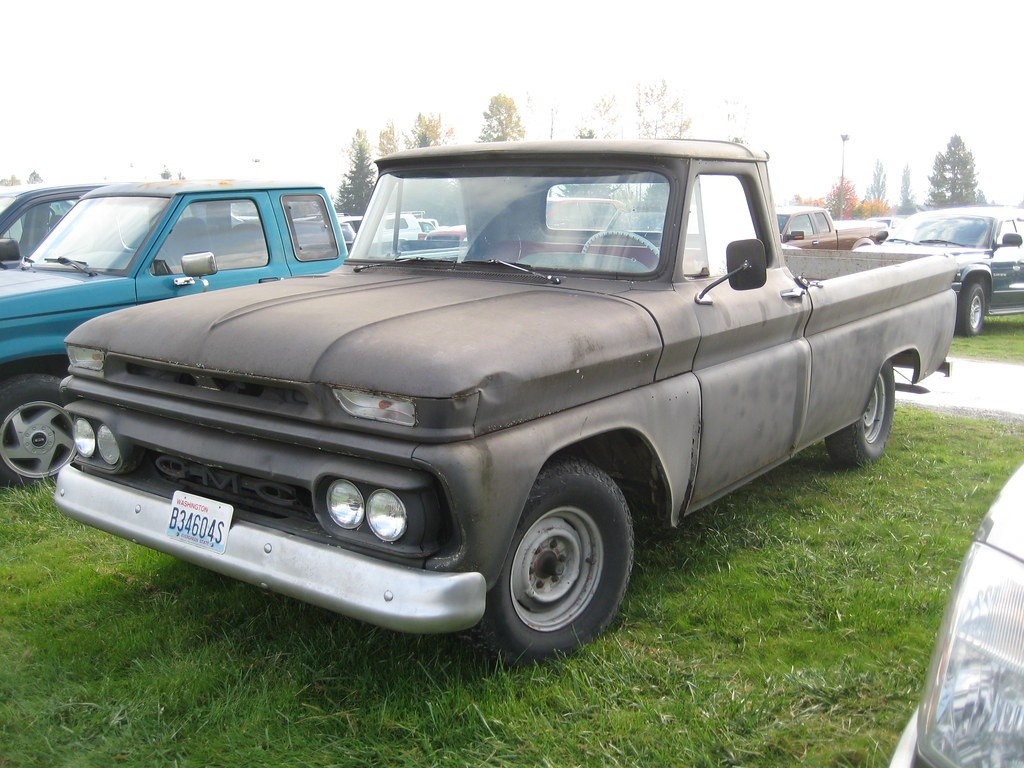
[486,238,704,272]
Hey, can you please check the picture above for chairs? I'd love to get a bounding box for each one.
[173,218,266,265]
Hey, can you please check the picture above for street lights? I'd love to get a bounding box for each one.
[840,133,852,216]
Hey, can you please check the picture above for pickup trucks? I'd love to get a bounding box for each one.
[1,138,957,666]
[773,205,888,252]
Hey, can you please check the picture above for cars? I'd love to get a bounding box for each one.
[854,205,1024,337]
